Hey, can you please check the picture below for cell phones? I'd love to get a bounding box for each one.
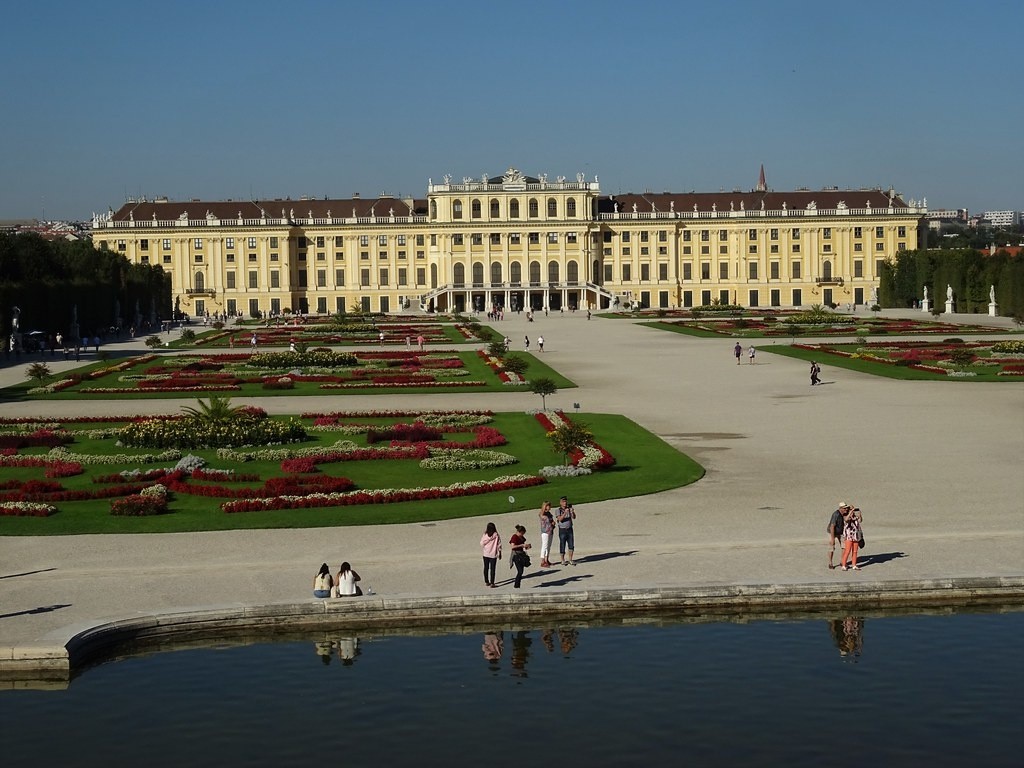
[568,504,572,509]
[854,509,860,511]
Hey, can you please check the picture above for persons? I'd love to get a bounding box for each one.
[539,501,556,567]
[524,336,530,351]
[733,341,755,365]
[480,523,502,588]
[379,332,424,350]
[989,284,996,303]
[22,317,170,362]
[488,311,503,322]
[526,307,591,322]
[313,562,363,598]
[827,502,863,571]
[428,167,599,185]
[847,302,856,311]
[509,524,532,589]
[810,361,821,385]
[923,285,928,302]
[537,336,544,353]
[316,637,362,666]
[482,629,575,681]
[556,498,576,565]
[946,283,954,303]
[613,196,927,215]
[504,336,512,351]
[229,331,258,352]
[92,206,414,223]
[204,309,227,327]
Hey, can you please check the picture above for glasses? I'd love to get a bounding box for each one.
[563,499,567,500]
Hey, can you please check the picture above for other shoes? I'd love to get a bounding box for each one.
[840,566,848,571]
[541,562,550,567]
[569,560,577,566]
[561,561,569,566]
[852,566,861,570]
[492,583,497,588]
[485,582,492,587]
[840,562,853,569]
[548,561,555,566]
[828,563,834,569]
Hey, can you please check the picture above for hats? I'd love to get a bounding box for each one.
[839,501,850,508]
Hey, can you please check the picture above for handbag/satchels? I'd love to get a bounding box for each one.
[858,538,870,549]
[516,553,531,567]
